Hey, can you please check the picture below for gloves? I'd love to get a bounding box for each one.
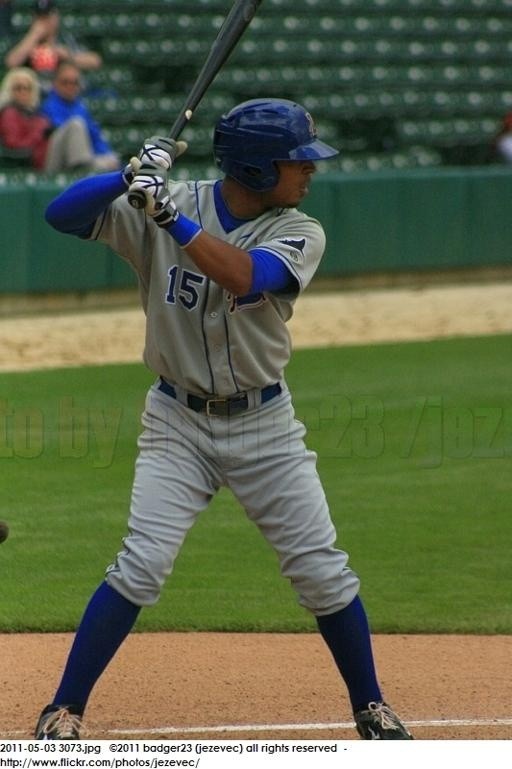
[121,135,188,228]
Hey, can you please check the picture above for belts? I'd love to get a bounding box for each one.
[157,379,283,417]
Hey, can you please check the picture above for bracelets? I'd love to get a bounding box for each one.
[168,213,204,249]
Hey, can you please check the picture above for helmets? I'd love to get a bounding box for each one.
[213,97,340,192]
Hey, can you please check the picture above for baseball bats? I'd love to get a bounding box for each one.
[126,0,260,208]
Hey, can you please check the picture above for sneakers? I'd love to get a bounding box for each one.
[354,702,415,740]
[34,704,82,739]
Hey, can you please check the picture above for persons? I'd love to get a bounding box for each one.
[42,68,126,169]
[4,1,106,69]
[1,66,95,176]
[34,99,411,741]
